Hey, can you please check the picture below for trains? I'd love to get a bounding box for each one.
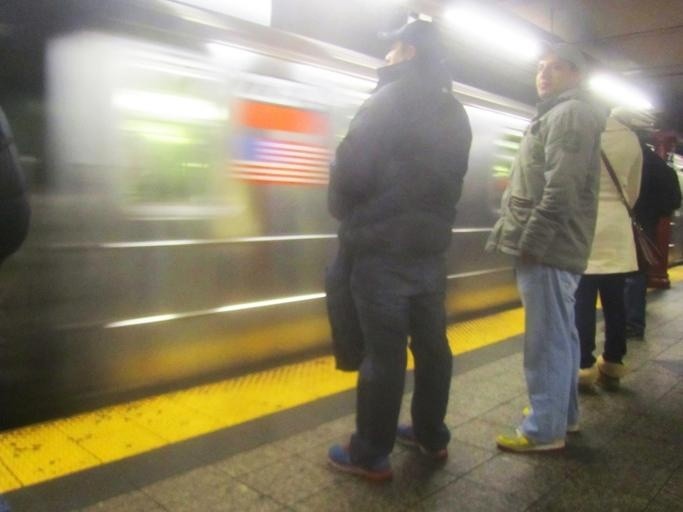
[0,1,683,431]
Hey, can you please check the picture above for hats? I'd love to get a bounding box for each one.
[379,20,440,48]
[533,39,588,74]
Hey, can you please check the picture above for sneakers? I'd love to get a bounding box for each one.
[395,423,449,462]
[494,428,567,453]
[522,406,580,433]
[327,444,394,481]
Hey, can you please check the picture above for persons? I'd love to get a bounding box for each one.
[327,19,472,483]
[575,79,643,392]
[484,50,600,452]
[624,126,650,341]
[1,106,31,261]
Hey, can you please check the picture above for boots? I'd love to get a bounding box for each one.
[576,366,598,390]
[591,354,628,393]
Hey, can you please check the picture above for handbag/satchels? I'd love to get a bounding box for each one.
[631,220,666,272]
[323,242,367,373]
